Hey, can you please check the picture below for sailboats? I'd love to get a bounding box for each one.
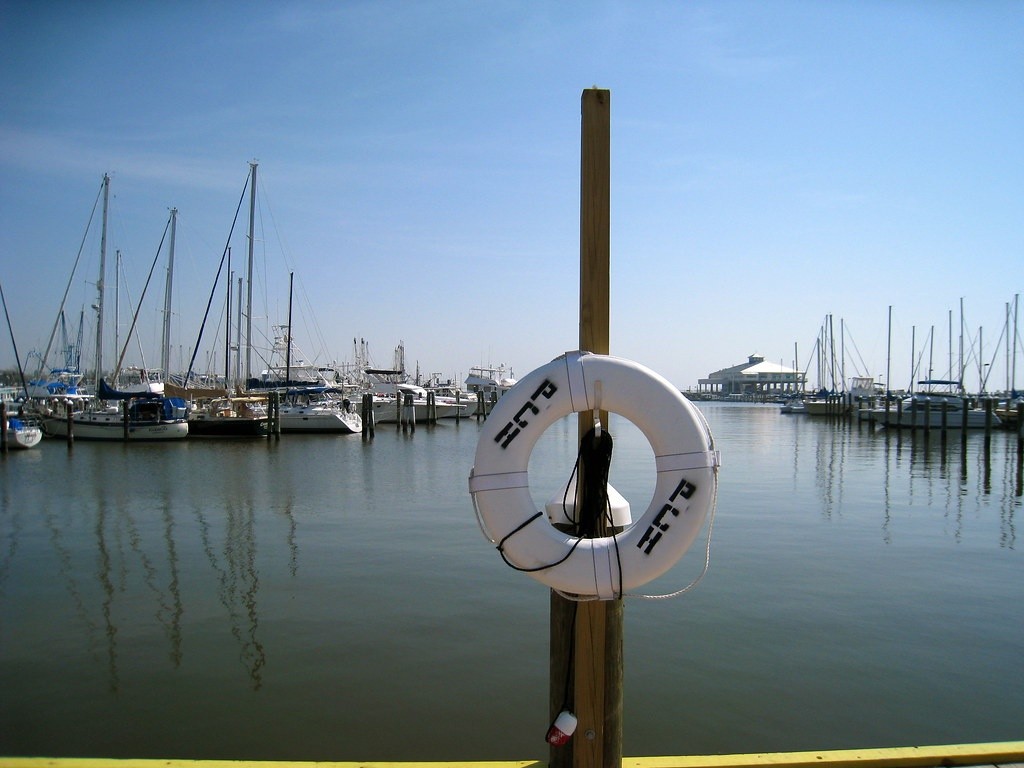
[29,162,363,441]
[778,289,1024,428]
[1,284,45,451]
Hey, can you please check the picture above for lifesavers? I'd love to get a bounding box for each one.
[468,350,721,601]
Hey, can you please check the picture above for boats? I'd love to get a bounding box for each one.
[343,334,522,424]
